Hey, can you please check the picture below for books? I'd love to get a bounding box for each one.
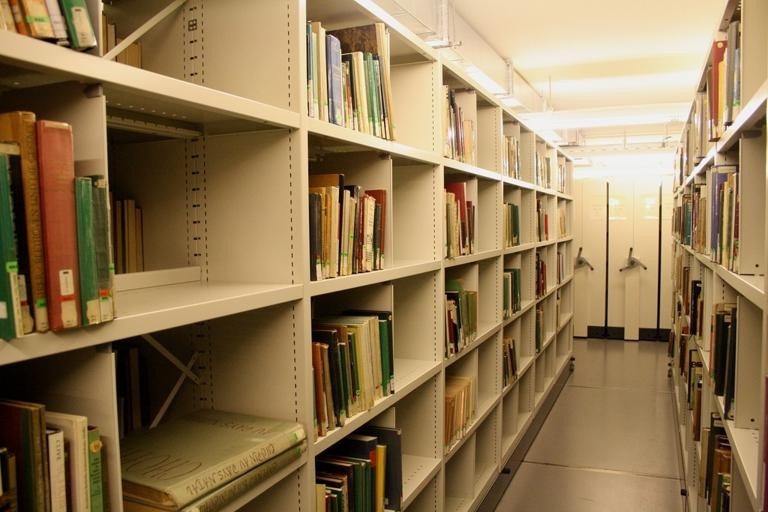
[444,278,477,358]
[504,268,522,320]
[309,174,387,281]
[536,305,546,354]
[0,398,104,512]
[537,198,548,242]
[0,110,115,341]
[315,426,401,512]
[443,181,475,259]
[558,253,563,285]
[536,252,547,300]
[102,13,142,71]
[0,0,99,50]
[113,197,148,274]
[312,309,394,435]
[129,346,142,433]
[503,136,520,179]
[445,375,476,454]
[119,407,308,512]
[557,164,565,192]
[557,289,562,328]
[668,0,768,511]
[504,202,520,248]
[537,151,551,189]
[444,84,474,166]
[306,20,397,141]
[503,338,518,388]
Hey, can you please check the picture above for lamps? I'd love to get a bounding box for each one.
[570,153,594,181]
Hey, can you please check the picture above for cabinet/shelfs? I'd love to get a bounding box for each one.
[666,0,768,512]
[0,0,577,512]
